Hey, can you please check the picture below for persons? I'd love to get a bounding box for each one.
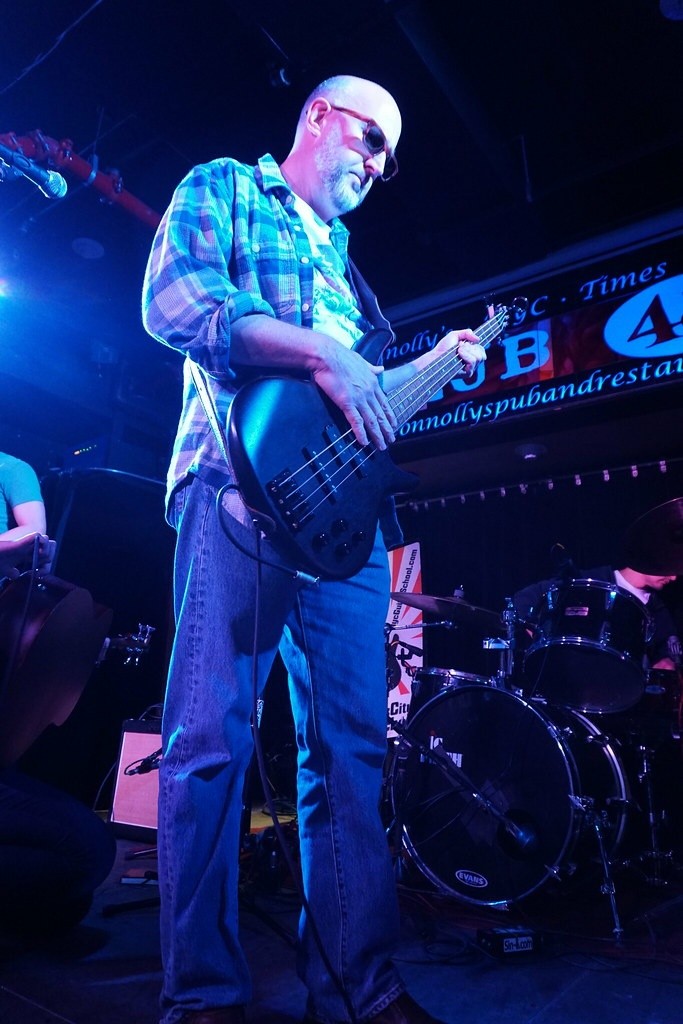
[140,73,491,1024]
[554,562,679,672]
[0,450,47,553]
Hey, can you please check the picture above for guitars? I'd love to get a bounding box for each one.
[222,300,514,583]
[0,569,158,773]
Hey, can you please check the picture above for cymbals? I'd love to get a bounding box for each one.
[390,587,503,628]
[617,495,682,578]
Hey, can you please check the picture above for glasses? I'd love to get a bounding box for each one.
[306,105,398,181]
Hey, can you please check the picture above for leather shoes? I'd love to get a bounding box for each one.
[303,996,446,1024]
[168,1002,246,1024]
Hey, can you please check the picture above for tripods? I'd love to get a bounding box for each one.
[608,730,683,897]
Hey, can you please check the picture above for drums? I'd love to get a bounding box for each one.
[387,677,629,916]
[403,666,498,743]
[521,577,653,717]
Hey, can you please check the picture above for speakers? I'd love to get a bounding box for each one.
[107,718,253,853]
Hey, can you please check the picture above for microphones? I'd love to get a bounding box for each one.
[127,759,161,776]
[515,824,538,853]
[0,144,67,198]
[557,543,577,580]
[397,640,424,657]
